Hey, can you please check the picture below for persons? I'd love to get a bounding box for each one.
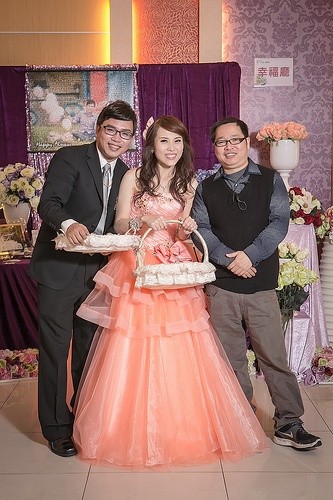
[29,99,138,459]
[70,97,102,143]
[188,118,324,452]
[86,112,208,462]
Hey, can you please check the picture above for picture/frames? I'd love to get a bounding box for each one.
[0,223,28,252]
[23,62,137,154]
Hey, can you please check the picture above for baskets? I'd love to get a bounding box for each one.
[134,220,215,289]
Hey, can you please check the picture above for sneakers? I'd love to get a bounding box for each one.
[273,420,322,449]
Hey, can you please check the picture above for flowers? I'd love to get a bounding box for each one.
[0,162,44,213]
[311,344,333,383]
[246,349,259,378]
[256,120,309,144]
[0,344,39,382]
[286,186,333,248]
[274,242,319,336]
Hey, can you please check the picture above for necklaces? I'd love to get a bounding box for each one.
[160,183,170,191]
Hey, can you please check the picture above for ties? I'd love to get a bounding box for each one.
[88,163,111,256]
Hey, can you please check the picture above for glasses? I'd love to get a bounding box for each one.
[213,138,246,147]
[99,124,135,140]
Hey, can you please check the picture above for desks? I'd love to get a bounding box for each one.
[322,376,328,386]
[0,256,41,349]
[278,222,324,383]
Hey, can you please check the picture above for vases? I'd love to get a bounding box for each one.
[268,139,300,193]
[2,202,31,227]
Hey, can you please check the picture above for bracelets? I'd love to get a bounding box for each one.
[130,218,144,232]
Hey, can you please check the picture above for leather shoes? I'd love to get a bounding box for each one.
[48,438,77,455]
